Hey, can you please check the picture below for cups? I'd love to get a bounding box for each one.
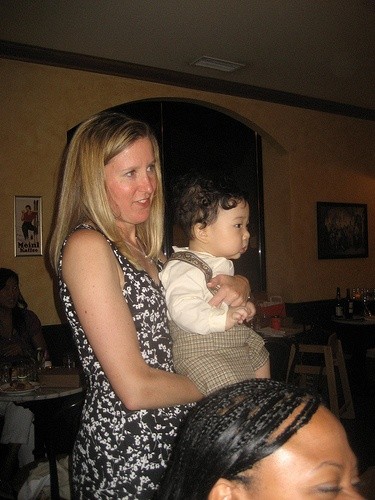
[63,352,76,369]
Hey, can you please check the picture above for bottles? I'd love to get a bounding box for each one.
[335,287,375,318]
[0,346,55,384]
[272,315,280,330]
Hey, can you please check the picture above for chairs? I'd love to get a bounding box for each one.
[289,334,355,419]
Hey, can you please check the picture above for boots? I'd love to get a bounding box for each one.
[0,443,21,500]
[23,461,36,480]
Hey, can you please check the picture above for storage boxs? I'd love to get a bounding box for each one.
[256,295,286,318]
[40,369,82,387]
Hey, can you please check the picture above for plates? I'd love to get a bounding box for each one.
[0,382,41,395]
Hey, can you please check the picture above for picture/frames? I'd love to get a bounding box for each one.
[317,201,369,259]
[14,195,43,257]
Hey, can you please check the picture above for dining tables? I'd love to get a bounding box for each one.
[0,386,85,465]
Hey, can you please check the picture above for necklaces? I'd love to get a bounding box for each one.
[126,237,148,259]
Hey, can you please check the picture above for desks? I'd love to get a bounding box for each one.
[332,315,375,325]
[259,325,314,361]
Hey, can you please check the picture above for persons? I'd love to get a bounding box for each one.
[152,379,365,500]
[325,224,361,252]
[21,205,38,241]
[158,175,271,397]
[49,112,251,500]
[0,268,49,355]
